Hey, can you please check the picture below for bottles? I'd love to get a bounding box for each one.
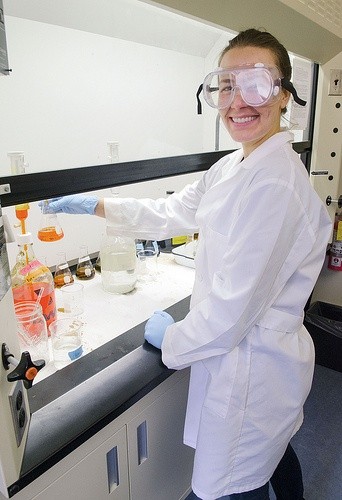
[10,232,58,337]
[75,244,96,281]
[37,199,64,243]
[14,301,49,368]
[54,251,75,290]
[95,250,102,274]
[99,232,137,295]
[328,240,342,270]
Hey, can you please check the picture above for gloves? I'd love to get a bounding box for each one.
[144,309,175,348]
[38,193,99,215]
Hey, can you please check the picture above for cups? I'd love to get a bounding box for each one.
[60,282,84,317]
[137,250,158,283]
[47,317,84,369]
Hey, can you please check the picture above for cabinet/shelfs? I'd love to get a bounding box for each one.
[9,366,196,500]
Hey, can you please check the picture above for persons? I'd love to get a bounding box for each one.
[38,29,330,500]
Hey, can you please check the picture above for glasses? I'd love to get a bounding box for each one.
[202,62,287,110]
